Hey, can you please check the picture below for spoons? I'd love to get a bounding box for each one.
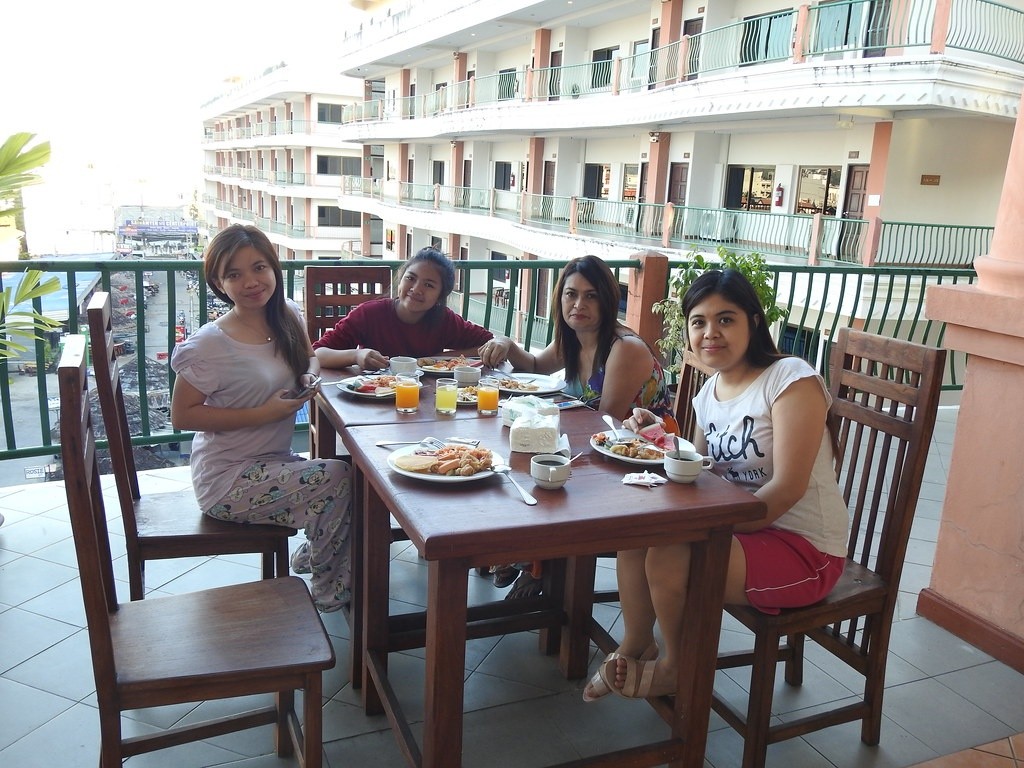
[490,464,537,505]
[601,414,633,445]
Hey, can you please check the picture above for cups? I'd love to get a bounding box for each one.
[386,356,418,375]
[454,368,482,388]
[435,378,459,415]
[664,450,714,483]
[530,454,572,490]
[478,378,500,416]
[396,372,419,412]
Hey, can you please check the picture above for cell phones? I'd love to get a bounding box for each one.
[556,400,584,409]
[280,377,321,400]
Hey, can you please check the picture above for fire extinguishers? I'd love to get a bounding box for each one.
[510,172,515,186]
[775,182,784,206]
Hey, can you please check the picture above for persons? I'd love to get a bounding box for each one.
[584,270,850,699]
[479,257,673,601]
[179,309,185,317]
[313,246,495,371]
[171,224,354,611]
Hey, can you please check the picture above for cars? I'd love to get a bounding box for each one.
[175,326,185,342]
[187,279,230,320]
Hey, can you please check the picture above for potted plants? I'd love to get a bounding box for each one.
[652,244,790,409]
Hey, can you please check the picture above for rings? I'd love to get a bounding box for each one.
[486,346,492,351]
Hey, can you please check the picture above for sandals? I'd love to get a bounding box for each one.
[605,657,679,700]
[582,639,660,702]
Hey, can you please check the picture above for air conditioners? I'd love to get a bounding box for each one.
[700,209,734,241]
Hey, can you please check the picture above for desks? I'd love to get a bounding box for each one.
[313,357,767,768]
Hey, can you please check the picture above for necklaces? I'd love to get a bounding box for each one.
[233,307,272,343]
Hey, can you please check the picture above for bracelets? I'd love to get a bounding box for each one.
[306,371,317,378]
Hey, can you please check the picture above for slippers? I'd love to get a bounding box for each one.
[488,562,519,589]
[505,570,542,601]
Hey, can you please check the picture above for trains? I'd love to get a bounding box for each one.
[116,219,199,236]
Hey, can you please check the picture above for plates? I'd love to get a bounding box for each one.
[414,357,485,376]
[380,366,424,377]
[387,443,504,483]
[457,388,477,406]
[337,375,424,398]
[498,373,566,393]
[589,429,696,465]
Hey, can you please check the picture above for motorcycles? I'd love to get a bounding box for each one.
[178,313,185,324]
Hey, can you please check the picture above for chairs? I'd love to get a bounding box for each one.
[88,293,297,602]
[492,287,510,308]
[673,338,717,443]
[59,334,335,768]
[711,327,947,768]
[304,266,393,459]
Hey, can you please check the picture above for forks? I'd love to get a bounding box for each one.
[491,368,537,383]
[376,437,446,450]
